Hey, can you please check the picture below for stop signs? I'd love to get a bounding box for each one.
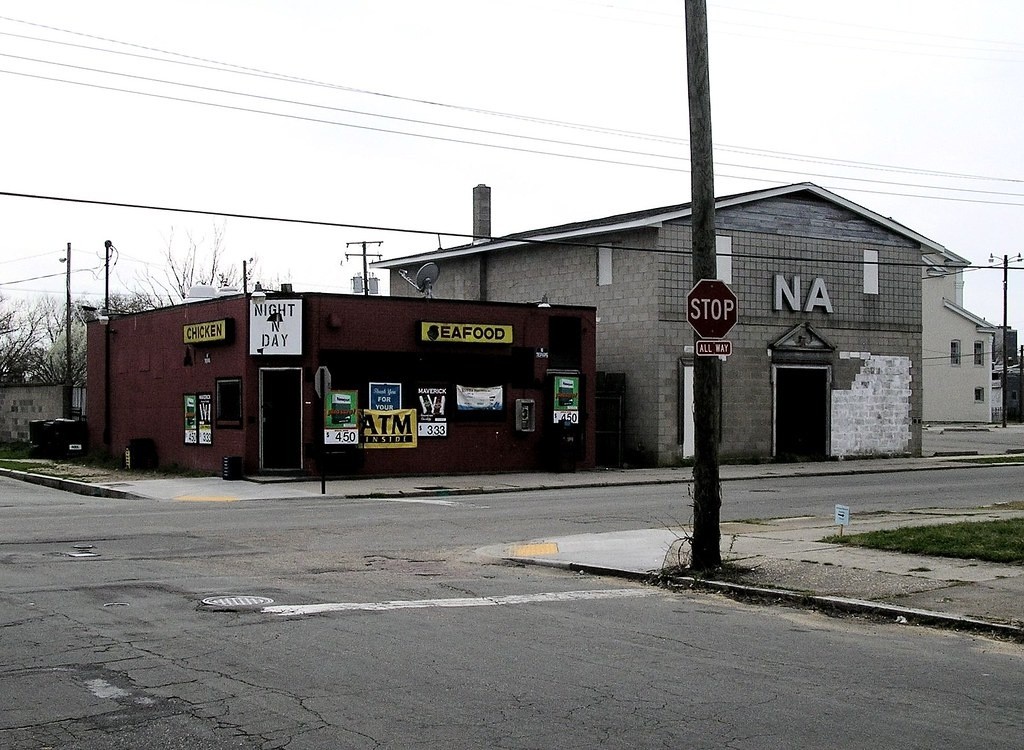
[686,278,739,340]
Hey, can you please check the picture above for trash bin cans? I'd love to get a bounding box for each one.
[221,454,244,482]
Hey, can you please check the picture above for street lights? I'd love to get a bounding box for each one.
[986,252,1024,427]
